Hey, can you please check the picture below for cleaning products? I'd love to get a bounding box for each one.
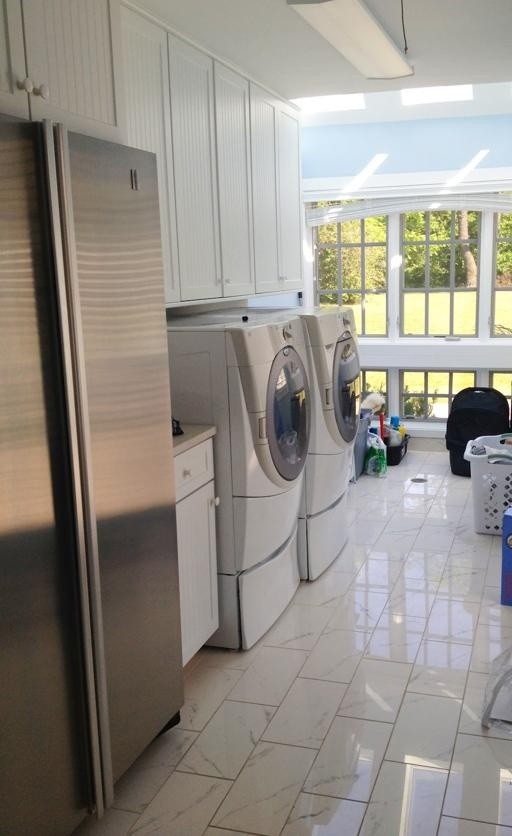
[366,432,388,477]
[389,429,402,445]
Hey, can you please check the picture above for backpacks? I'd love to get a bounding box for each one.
[447,387,509,476]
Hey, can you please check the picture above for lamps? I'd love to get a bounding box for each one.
[288,0,417,82]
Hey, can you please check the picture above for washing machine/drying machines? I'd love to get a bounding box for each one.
[165,314,312,652]
[270,307,364,583]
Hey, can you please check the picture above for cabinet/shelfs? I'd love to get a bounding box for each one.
[129,6,307,308]
[0,0,129,149]
[172,437,219,669]
